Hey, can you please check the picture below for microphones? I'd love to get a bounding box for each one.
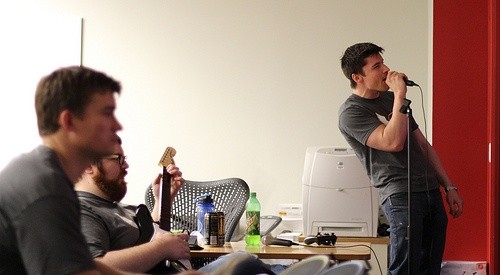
[261,235,298,246]
[403,78,420,87]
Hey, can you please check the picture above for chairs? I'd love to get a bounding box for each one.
[144,177,249,241]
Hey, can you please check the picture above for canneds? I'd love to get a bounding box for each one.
[204,211,225,247]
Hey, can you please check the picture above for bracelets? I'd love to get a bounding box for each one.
[445,185,457,191]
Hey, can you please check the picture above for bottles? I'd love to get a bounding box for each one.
[245,192,261,245]
[195,194,215,246]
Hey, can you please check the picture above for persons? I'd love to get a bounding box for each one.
[74,136,286,275]
[0,66,204,275]
[337,42,464,275]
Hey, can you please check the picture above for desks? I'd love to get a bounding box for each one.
[190,241,372,272]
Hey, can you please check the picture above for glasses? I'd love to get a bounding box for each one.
[99,152,125,166]
